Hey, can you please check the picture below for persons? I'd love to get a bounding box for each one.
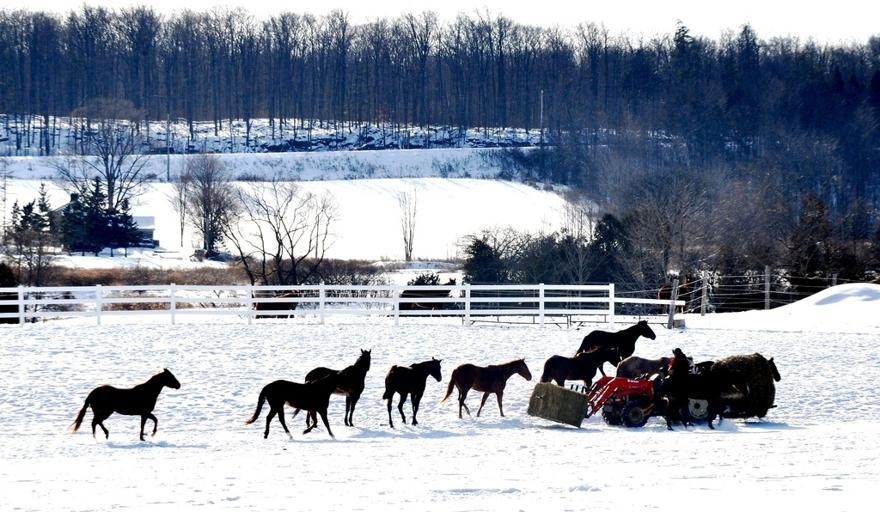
[665,348,690,430]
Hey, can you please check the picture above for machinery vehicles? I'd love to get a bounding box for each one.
[567,355,781,434]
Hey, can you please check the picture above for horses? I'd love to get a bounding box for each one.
[440,357,532,420]
[574,320,656,376]
[67,368,181,442]
[659,357,780,430]
[540,345,623,391]
[616,355,671,380]
[293,348,372,427]
[382,356,443,429]
[243,371,348,442]
